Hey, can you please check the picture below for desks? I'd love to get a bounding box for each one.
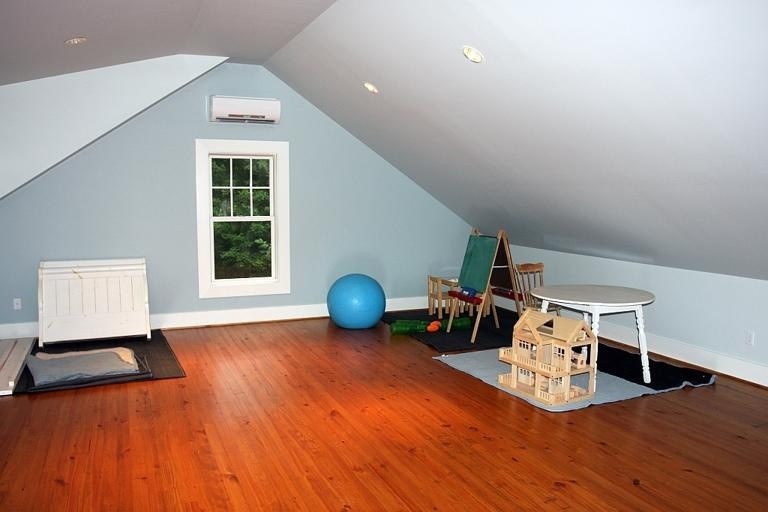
[529,284,655,385]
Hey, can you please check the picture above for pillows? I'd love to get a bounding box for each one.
[26,346,145,387]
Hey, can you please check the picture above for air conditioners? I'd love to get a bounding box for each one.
[207,93,282,128]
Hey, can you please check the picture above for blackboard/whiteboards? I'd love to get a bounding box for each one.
[457,234,501,293]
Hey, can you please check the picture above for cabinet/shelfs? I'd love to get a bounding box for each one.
[34,259,153,348]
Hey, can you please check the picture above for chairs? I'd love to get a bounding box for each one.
[512,262,559,319]
[428,276,459,319]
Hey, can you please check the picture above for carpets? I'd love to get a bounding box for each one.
[382,304,522,353]
[12,327,185,396]
[431,343,716,411]
